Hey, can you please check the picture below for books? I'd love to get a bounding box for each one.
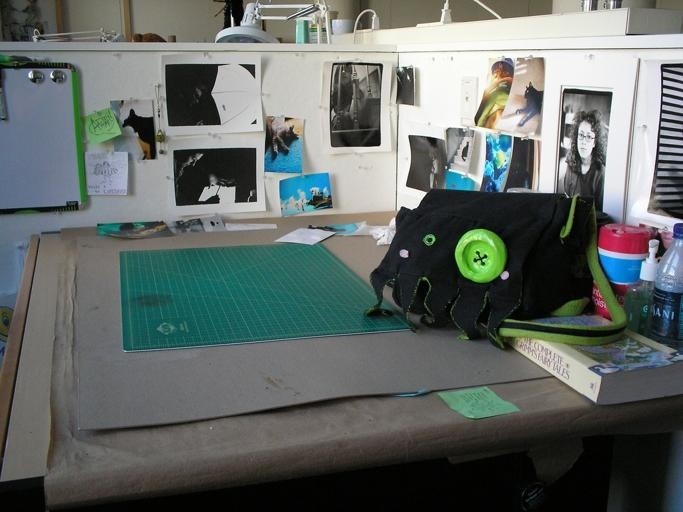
[497,314,683,407]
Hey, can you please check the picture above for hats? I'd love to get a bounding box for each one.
[491,58,516,76]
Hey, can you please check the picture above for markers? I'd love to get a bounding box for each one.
[0,70,7,121]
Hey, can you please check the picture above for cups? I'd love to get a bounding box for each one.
[307,10,338,43]
[592,223,653,320]
[332,19,354,34]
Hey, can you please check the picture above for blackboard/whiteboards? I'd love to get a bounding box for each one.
[0,63,88,215]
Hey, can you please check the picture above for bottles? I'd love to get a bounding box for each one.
[649,222,683,354]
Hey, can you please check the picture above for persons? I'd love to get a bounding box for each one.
[554,105,604,214]
[330,63,366,148]
[474,61,513,132]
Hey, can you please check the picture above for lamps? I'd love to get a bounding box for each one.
[214,0,330,44]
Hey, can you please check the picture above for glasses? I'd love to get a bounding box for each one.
[577,132,596,141]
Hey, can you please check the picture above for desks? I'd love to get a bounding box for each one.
[1,209,682,512]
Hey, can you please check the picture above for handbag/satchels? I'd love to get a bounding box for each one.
[362,188,630,349]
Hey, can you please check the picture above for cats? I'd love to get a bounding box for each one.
[515,81,543,126]
[271,116,299,156]
